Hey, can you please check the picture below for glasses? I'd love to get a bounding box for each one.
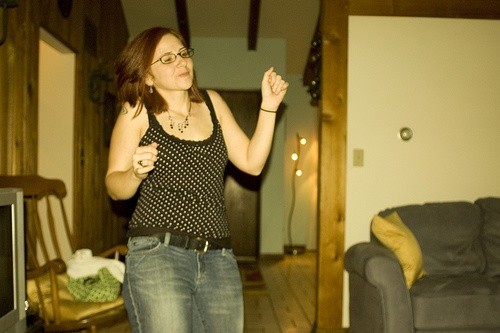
[151,47,194,65]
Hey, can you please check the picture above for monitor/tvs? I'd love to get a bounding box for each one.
[0,187,27,333]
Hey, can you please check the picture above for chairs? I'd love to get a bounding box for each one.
[0,175,128,333]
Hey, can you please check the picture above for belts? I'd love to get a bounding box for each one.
[131,229,231,252]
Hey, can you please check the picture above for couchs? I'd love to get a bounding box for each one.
[343,196,500,333]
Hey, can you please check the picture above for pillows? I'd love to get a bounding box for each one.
[372,211,428,290]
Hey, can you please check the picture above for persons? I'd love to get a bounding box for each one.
[104,27,289,333]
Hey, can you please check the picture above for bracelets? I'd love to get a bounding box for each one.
[260,107,277,113]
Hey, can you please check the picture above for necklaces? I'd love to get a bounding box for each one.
[167,100,191,133]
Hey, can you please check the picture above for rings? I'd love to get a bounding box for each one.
[138,160,142,164]
